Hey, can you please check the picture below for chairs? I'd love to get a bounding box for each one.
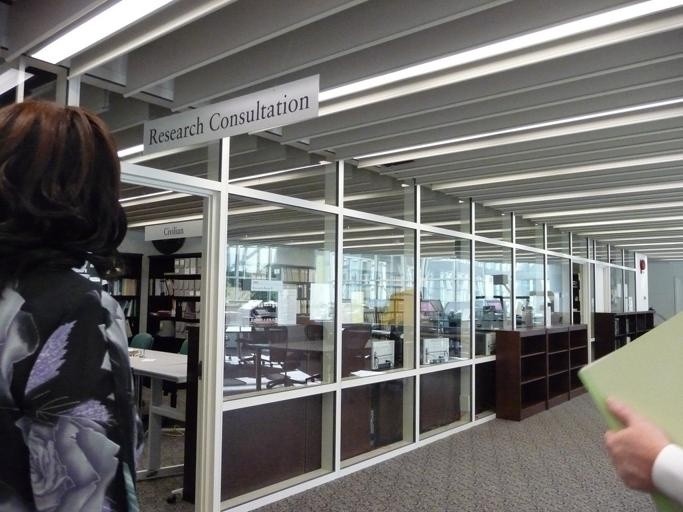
[235,318,371,384]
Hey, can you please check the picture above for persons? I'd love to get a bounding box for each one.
[0,98,151,511]
[601,398,682,504]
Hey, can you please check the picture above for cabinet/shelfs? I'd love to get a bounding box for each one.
[268,264,319,317]
[591,311,656,360]
[495,323,590,422]
[105,251,200,354]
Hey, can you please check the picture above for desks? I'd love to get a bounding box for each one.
[121,344,186,481]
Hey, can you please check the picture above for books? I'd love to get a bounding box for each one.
[343,261,401,324]
[349,369,382,377]
[105,278,137,338]
[422,337,449,366]
[153,257,316,392]
[485,333,497,355]
[577,307,683,512]
[368,339,395,369]
